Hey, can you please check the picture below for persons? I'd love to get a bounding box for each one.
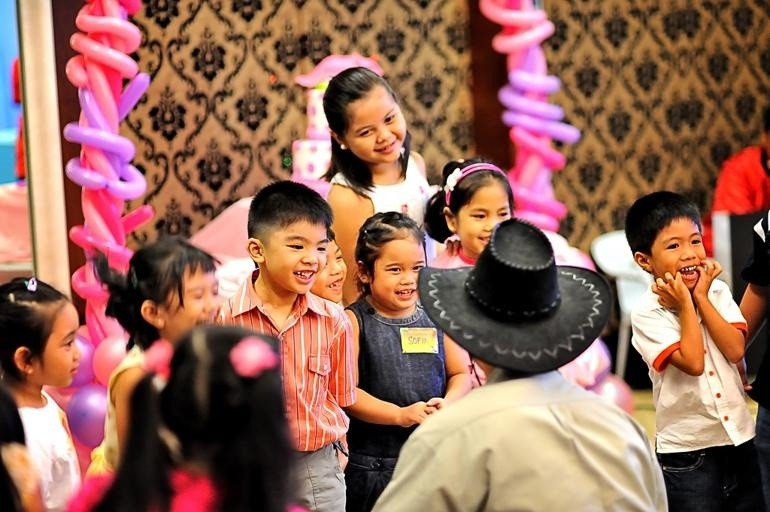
[321,63,462,311]
[738,208,770,511]
[703,106,770,254]
[0,388,46,512]
[310,225,351,308]
[346,211,476,512]
[368,217,670,511]
[623,191,770,512]
[1,275,83,511]
[61,324,313,511]
[422,156,518,389]
[213,179,358,511]
[85,235,224,480]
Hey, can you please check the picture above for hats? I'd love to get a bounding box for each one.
[417,219,612,376]
